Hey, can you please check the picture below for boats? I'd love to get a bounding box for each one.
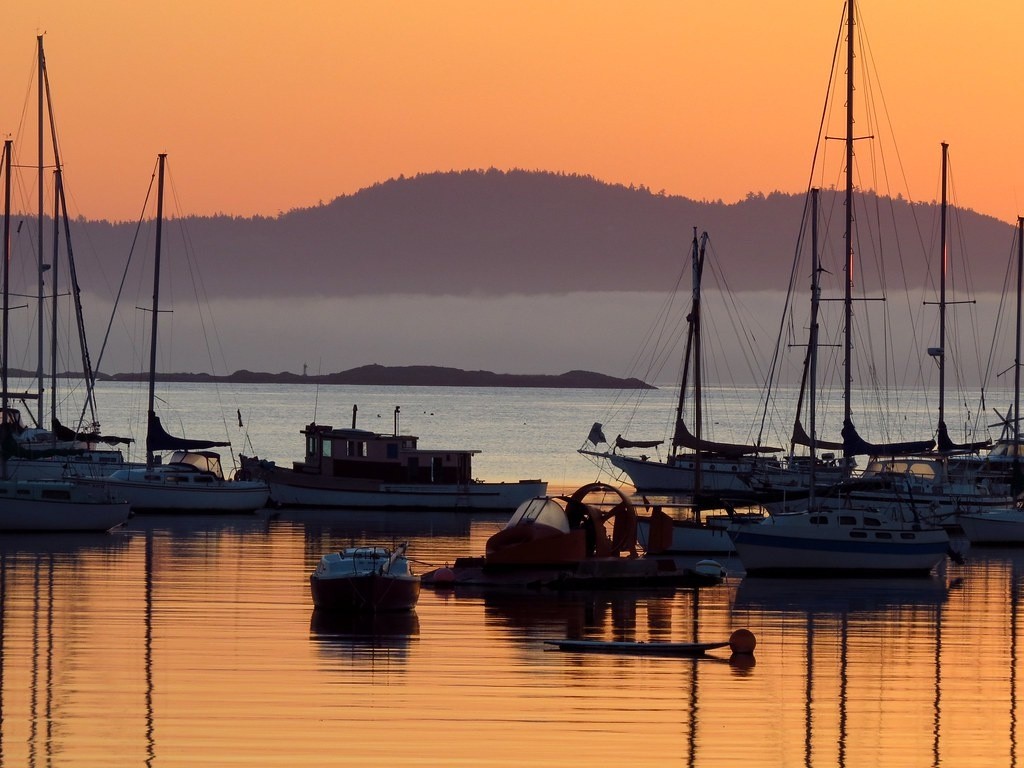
[109,155,271,514]
[421,483,682,595]
[578,0,1024,580]
[0,35,130,531]
[235,405,547,513]
[309,537,421,614]
